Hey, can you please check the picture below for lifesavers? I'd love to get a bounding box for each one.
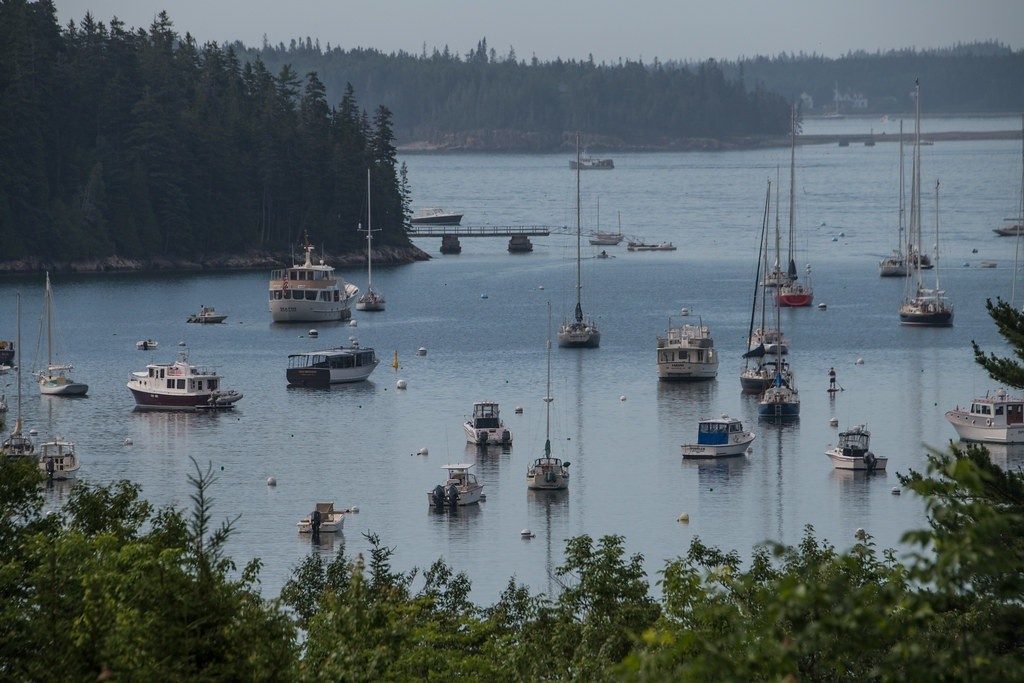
[175,370,181,376]
[986,418,991,426]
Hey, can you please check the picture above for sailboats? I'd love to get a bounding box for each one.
[356,168,387,311]
[556,134,602,351]
[721,104,814,420]
[588,196,625,245]
[0,294,39,459]
[41,271,91,397]
[528,300,572,492]
[889,76,957,327]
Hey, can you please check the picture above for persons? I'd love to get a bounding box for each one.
[44,457,54,490]
[281,282,288,296]
[369,291,376,304]
[828,367,836,388]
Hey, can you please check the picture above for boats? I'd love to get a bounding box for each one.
[834,422,892,472]
[944,387,1024,445]
[284,340,381,386]
[992,217,1024,236]
[625,238,678,253]
[36,396,84,481]
[410,206,463,225]
[267,228,360,325]
[656,307,721,380]
[464,401,514,446]
[295,501,346,535]
[426,423,488,509]
[135,337,158,350]
[185,304,229,323]
[0,340,16,363]
[126,345,244,414]
[569,149,616,170]
[681,414,757,460]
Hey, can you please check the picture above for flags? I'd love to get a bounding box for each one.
[392,350,399,369]
